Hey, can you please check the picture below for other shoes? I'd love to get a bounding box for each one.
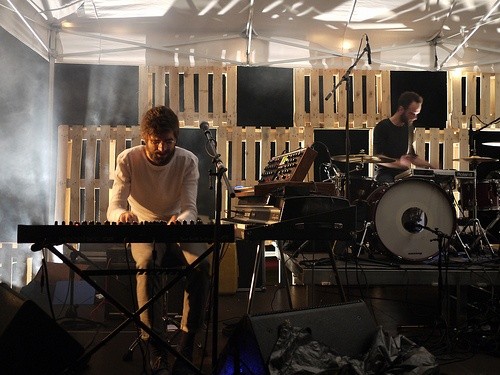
[178,331,193,362]
[145,340,170,375]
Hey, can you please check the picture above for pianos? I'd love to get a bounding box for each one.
[212,146,358,314]
[17,216,235,375]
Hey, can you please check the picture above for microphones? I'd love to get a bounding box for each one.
[199,121,213,143]
[366,41,372,64]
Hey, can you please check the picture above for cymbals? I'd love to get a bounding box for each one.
[482,141,500,147]
[330,154,396,164]
[460,156,494,164]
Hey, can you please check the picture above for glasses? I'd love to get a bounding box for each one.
[148,135,176,146]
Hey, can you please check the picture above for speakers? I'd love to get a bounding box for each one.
[0,259,128,375]
[212,298,383,375]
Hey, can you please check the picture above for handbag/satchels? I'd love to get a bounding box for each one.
[267,320,370,375]
[366,328,442,375]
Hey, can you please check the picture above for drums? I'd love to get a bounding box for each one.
[460,180,490,211]
[340,177,377,206]
[485,179,500,210]
[363,178,457,265]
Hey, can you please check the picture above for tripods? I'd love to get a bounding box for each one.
[451,159,498,256]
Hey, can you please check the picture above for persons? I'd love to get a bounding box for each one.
[372,91,433,184]
[106,106,211,375]
